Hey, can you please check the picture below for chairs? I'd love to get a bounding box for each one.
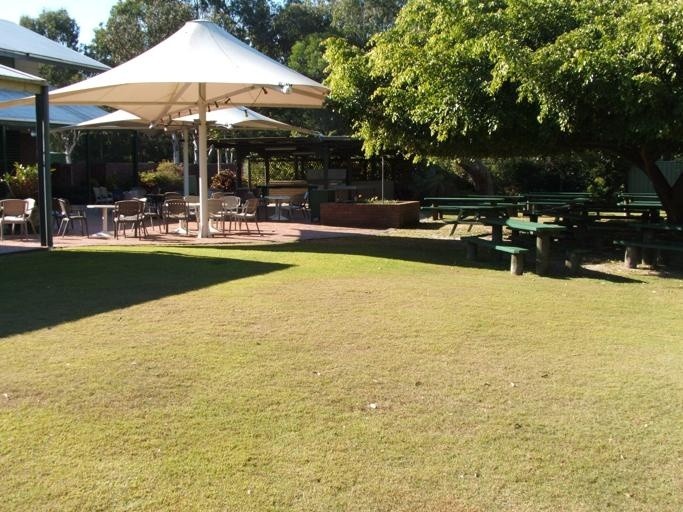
[0,197,39,243]
[52,191,309,239]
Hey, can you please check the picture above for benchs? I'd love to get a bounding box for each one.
[565,247,592,272]
[462,235,531,276]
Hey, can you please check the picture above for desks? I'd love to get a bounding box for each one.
[420,191,663,236]
[478,218,567,274]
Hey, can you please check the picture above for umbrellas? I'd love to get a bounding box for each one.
[0,19,332,241]
[73,104,293,234]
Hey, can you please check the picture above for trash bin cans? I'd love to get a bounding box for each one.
[310,189,336,223]
